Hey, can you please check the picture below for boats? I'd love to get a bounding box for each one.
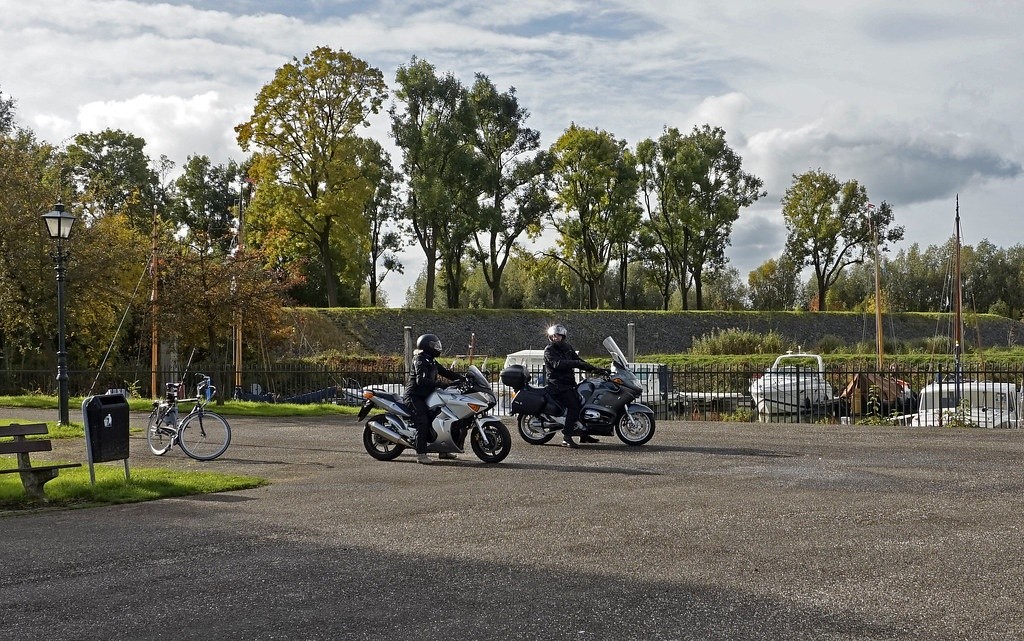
[749,345,834,416]
[340,384,409,406]
[488,349,742,417]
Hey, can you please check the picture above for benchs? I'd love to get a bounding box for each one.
[0,423,82,499]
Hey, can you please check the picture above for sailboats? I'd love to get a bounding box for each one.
[909,194,1021,429]
[841,204,918,414]
[79,184,348,405]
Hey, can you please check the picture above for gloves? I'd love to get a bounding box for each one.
[455,373,461,380]
[439,381,449,390]
[579,361,588,371]
[598,368,612,377]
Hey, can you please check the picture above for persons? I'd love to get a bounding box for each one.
[402,334,465,464]
[543,324,611,447]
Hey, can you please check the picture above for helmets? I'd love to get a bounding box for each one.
[416,333,442,357]
[547,324,567,346]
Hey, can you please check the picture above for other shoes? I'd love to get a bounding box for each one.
[417,456,432,464]
[439,452,457,459]
[580,435,600,443]
[562,439,578,448]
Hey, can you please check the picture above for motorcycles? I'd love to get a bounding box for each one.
[358,364,512,464]
[499,335,655,447]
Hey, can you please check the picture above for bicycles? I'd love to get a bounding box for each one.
[146,373,231,460]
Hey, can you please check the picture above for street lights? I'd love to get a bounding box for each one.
[41,198,79,429]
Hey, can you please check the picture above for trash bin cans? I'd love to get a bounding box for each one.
[79,392,132,485]
